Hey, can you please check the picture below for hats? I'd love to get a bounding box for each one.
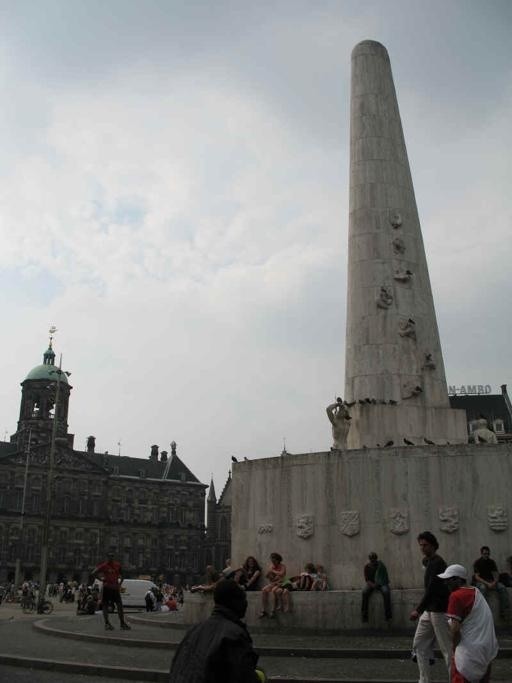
[436,564,468,579]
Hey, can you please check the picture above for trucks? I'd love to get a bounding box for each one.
[92,577,161,607]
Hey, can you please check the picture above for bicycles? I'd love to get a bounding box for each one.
[23,595,54,615]
[0,586,14,602]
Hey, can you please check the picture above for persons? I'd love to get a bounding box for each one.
[472,545,501,637]
[225,556,262,591]
[299,560,313,590]
[324,396,352,450]
[471,416,499,445]
[89,551,133,631]
[275,574,301,613]
[257,551,286,620]
[167,576,266,682]
[407,529,456,683]
[359,551,395,624]
[497,553,512,588]
[310,563,331,592]
[1,556,233,618]
[435,562,499,682]
